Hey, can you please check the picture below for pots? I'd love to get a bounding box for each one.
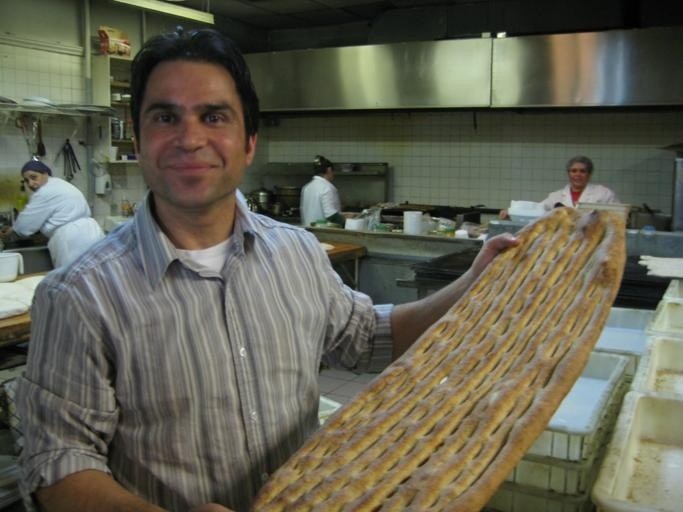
[250,187,274,212]
[274,182,300,212]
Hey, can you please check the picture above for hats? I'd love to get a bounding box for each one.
[22,161,52,177]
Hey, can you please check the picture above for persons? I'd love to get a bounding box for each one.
[0,160,105,268]
[500,155,619,222]
[16,23,520,512]
[298,154,358,232]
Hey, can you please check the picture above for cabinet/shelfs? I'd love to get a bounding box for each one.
[90,54,139,165]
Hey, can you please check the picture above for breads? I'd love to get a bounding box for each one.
[249,207,626,512]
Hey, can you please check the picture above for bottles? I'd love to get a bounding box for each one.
[112,117,134,141]
[120,195,131,216]
[110,199,118,216]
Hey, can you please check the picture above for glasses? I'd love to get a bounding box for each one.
[567,167,588,174]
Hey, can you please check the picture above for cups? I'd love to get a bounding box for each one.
[111,93,122,101]
[120,94,131,102]
[111,146,120,161]
